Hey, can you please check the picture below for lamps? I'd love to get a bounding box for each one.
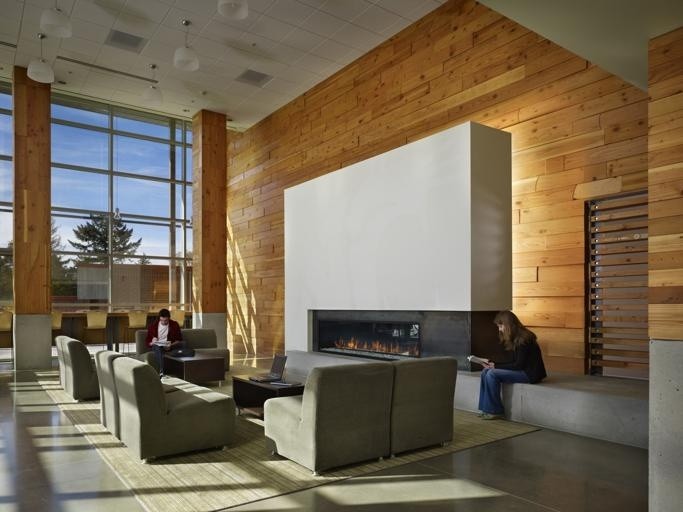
[214,1,249,20]
[26,32,55,83]
[173,19,200,72]
[141,62,164,108]
[38,1,73,40]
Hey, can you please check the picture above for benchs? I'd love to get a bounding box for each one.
[470,367,647,453]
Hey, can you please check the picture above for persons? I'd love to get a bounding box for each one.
[474,310,545,420]
[144,308,183,378]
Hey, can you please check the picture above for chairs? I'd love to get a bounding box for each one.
[53,308,191,400]
[262,360,395,477]
[95,347,198,441]
[389,355,460,459]
[112,355,235,464]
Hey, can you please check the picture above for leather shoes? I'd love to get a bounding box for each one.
[477,411,486,417]
[481,414,505,420]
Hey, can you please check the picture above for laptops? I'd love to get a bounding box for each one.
[249,353,288,382]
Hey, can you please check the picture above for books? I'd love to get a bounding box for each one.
[148,339,172,348]
[464,354,494,369]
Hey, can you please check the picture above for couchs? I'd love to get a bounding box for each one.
[134,327,230,371]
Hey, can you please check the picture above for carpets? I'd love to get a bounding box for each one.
[32,368,545,512]
